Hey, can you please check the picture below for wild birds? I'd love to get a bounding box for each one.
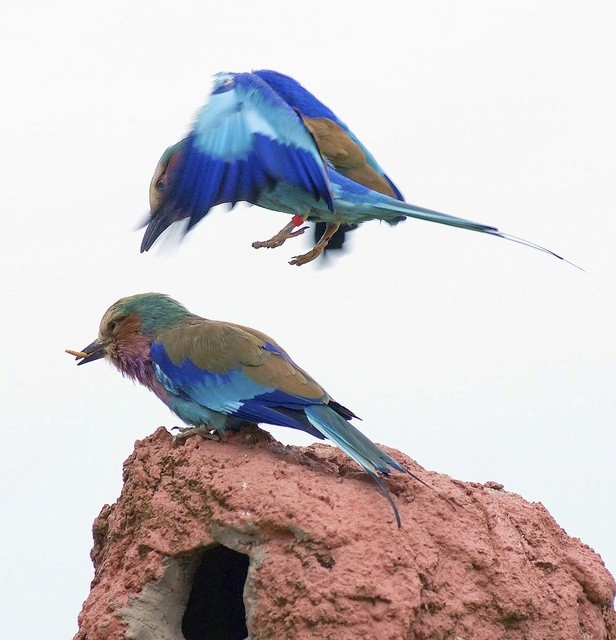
[63,290,462,532]
[131,66,590,269]
